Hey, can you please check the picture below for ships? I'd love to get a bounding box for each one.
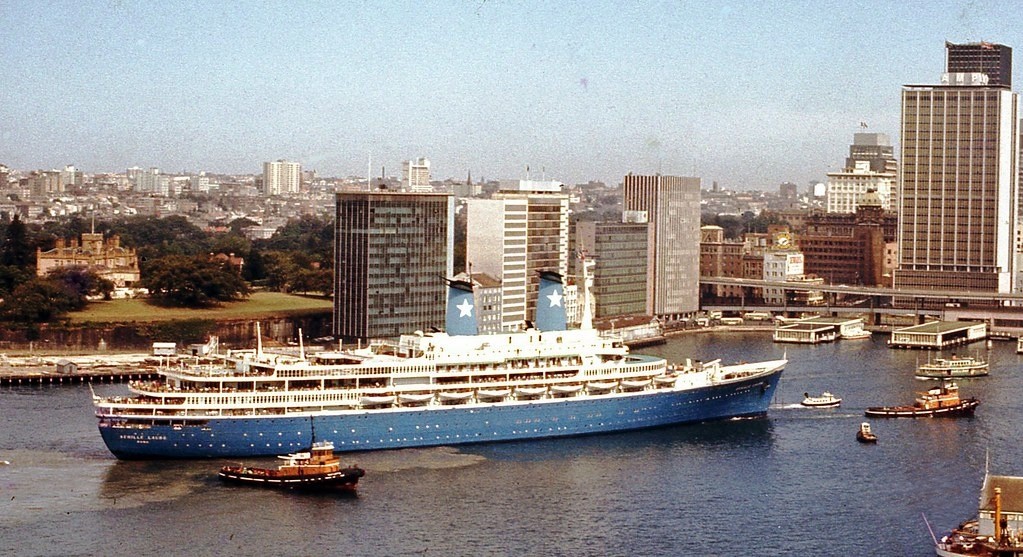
[88,232,788,463]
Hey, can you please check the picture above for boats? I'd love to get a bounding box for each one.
[840,326,872,340]
[620,379,653,390]
[399,393,435,403]
[478,390,512,399]
[360,396,397,405]
[653,376,676,384]
[915,350,990,380]
[922,487,1023,557]
[587,381,619,392]
[801,391,842,409]
[551,385,584,395]
[438,391,476,402]
[863,382,981,419]
[856,422,879,443]
[217,415,365,497]
[514,386,549,397]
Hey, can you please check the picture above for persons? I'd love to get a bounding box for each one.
[1000,519,1009,533]
